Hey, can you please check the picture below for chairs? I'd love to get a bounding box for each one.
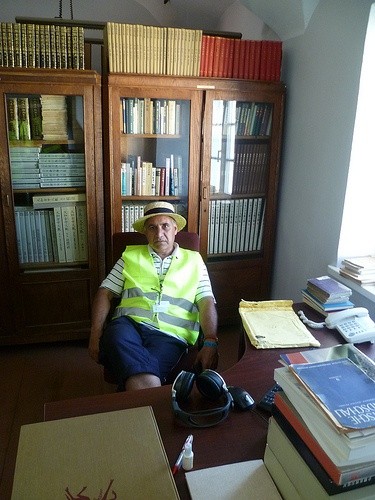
[98,231,219,392]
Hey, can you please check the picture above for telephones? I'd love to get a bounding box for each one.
[325,307,375,344]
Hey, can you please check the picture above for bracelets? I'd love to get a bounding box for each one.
[202,335,218,347]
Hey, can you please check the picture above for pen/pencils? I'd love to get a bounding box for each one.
[171,434,193,475]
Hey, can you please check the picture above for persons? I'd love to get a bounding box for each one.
[88,202,218,392]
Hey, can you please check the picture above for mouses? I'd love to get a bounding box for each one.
[227,386,255,411]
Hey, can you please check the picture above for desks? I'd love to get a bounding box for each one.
[44,301,375,500]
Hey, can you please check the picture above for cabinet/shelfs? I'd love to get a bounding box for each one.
[103,72,285,326]
[0,67,104,346]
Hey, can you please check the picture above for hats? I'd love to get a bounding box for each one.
[132,202,187,234]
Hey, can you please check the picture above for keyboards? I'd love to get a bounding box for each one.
[259,383,282,414]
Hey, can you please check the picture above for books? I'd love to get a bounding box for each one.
[207,197,265,254]
[120,98,190,135]
[301,275,354,317]
[261,341,375,500]
[0,22,85,70]
[222,140,271,194]
[103,21,283,82]
[223,102,273,136]
[120,153,188,197]
[14,193,88,263]
[339,256,375,287]
[6,92,86,189]
[122,200,187,232]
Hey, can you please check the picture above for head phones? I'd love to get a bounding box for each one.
[171,369,234,429]
